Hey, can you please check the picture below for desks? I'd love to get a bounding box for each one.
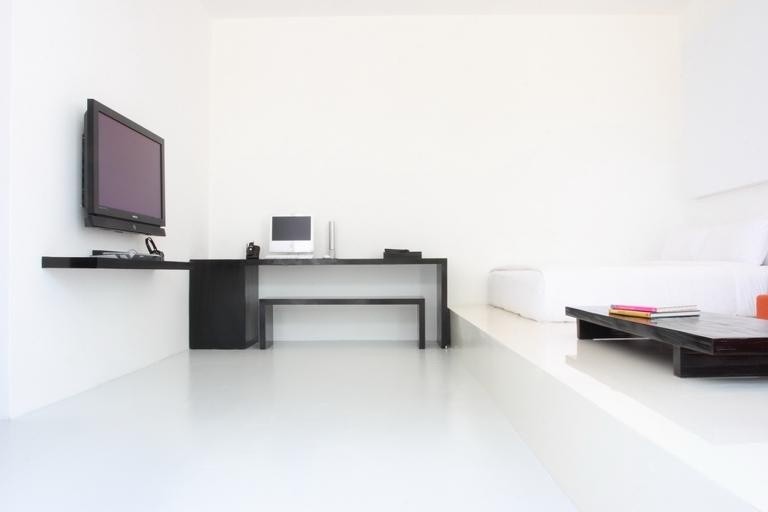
[190,258,448,349]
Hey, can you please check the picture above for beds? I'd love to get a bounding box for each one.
[488,180,768,322]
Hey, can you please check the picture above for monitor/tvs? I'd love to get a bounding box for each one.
[83,97,167,237]
[269,215,311,254]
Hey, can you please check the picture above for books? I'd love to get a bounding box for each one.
[609,302,701,318]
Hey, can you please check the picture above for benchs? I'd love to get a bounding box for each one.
[259,298,426,348]
